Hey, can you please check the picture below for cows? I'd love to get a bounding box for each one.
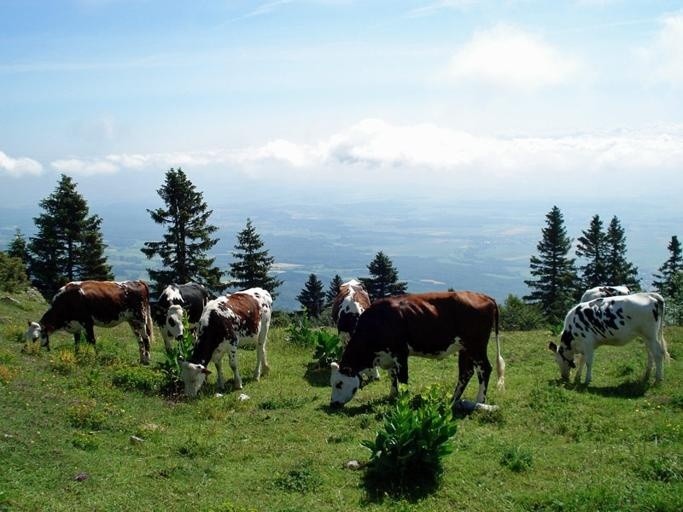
[155,281,217,376]
[331,279,381,382]
[580,285,631,303]
[25,279,154,365]
[548,292,671,390]
[328,290,506,405]
[178,286,273,400]
